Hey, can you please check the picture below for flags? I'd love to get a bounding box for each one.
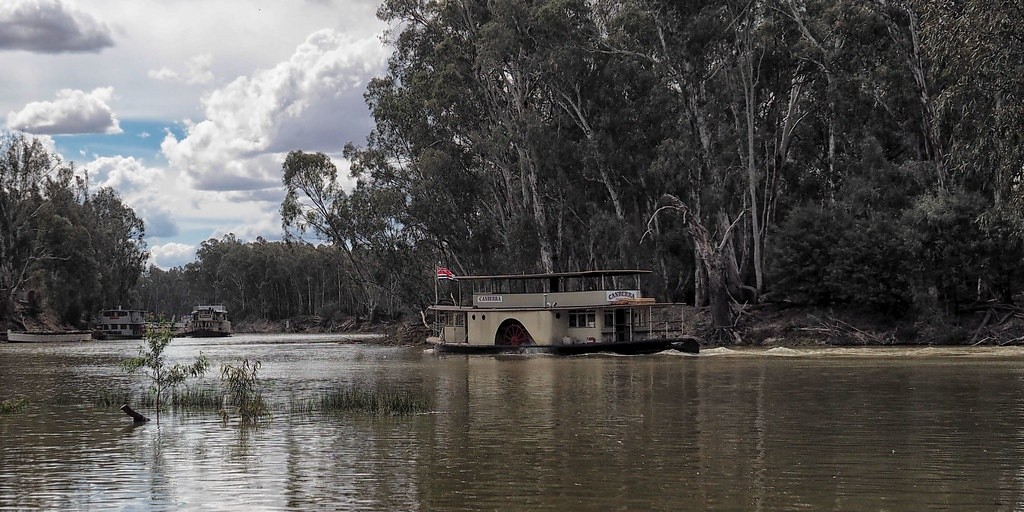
[437,267,453,277]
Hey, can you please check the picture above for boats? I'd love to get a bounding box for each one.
[417,263,701,355]
[90,305,148,340]
[184,304,233,336]
